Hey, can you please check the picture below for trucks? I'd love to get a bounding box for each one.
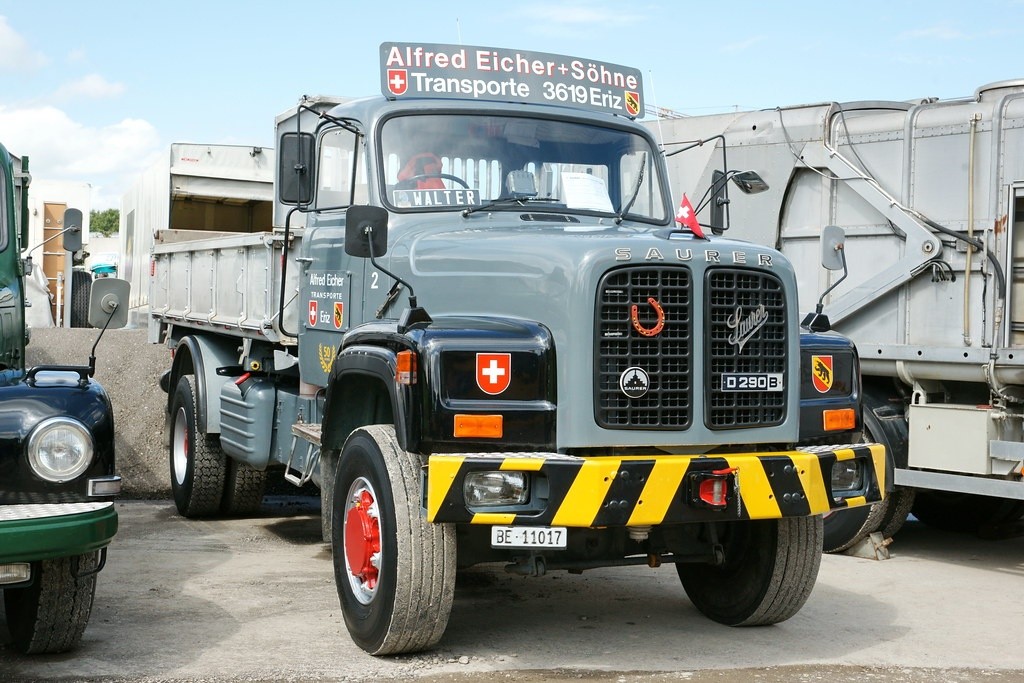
[144,95,888,662]
[559,78,1024,555]
[0,141,132,657]
